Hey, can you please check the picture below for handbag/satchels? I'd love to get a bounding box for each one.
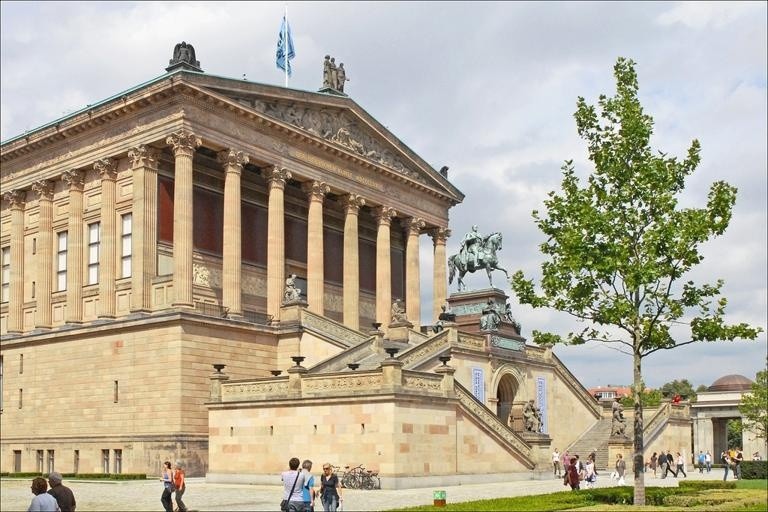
[280,501,288,510]
[168,486,175,492]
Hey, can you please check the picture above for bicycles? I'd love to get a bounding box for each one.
[331,463,381,492]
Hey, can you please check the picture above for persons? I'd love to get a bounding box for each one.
[551,448,762,491]
[610,404,626,436]
[47,472,76,512]
[27,477,61,512]
[432,304,450,333]
[466,225,482,267]
[300,460,314,512]
[323,55,350,92]
[316,463,343,512]
[522,400,541,433]
[160,462,173,512]
[506,303,521,330]
[480,298,500,332]
[612,396,625,418]
[392,299,407,320]
[174,464,187,512]
[286,275,301,300]
[281,458,305,512]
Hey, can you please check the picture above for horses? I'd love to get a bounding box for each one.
[448,232,511,293]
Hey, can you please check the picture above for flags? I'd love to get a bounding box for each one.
[276,16,295,76]
